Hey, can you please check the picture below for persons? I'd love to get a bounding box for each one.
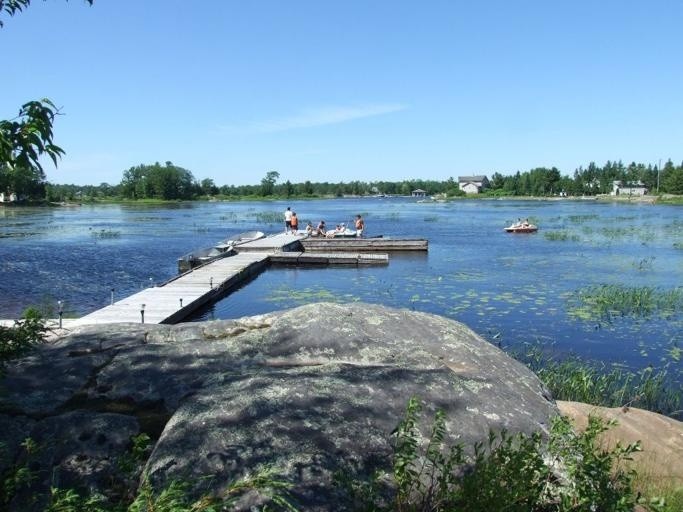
[520,219,528,228]
[510,218,521,228]
[283,207,293,235]
[352,214,364,238]
[314,220,347,239]
[304,222,314,235]
[288,212,298,234]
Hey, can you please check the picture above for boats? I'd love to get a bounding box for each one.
[326,230,357,237]
[503,223,537,232]
[215,231,264,246]
[176,243,234,271]
